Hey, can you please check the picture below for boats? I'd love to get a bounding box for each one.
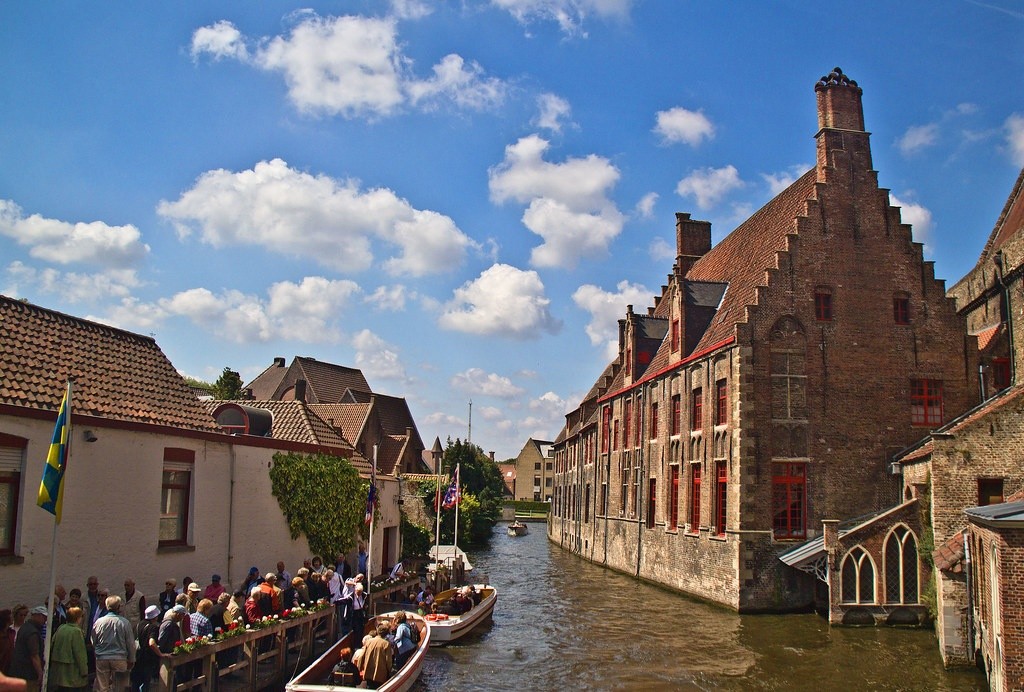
[508,520,528,536]
[285,610,431,692]
[418,583,497,647]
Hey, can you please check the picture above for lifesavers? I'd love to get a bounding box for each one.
[425,614,449,621]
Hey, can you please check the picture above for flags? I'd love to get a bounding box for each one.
[36,390,73,526]
[364,446,378,526]
[434,466,461,512]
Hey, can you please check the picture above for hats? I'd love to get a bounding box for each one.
[211,574,221,581]
[144,605,160,619]
[248,567,258,575]
[188,583,202,592]
[31,606,48,616]
[173,605,188,614]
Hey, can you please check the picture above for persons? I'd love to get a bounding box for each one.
[330,573,368,649]
[447,585,480,615]
[241,553,352,624]
[0,575,146,692]
[389,558,410,583]
[327,611,418,691]
[130,574,246,692]
[408,585,438,616]
[356,544,369,583]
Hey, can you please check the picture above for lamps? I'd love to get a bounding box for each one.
[396,499,404,505]
[892,462,901,475]
[84,430,98,443]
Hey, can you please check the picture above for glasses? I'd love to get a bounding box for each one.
[165,582,172,585]
[98,594,106,597]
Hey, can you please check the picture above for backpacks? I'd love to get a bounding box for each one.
[400,623,421,645]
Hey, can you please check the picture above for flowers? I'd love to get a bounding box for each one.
[253,614,279,630]
[309,596,332,612]
[173,633,212,655]
[282,603,307,620]
[214,616,250,640]
[370,570,417,592]
